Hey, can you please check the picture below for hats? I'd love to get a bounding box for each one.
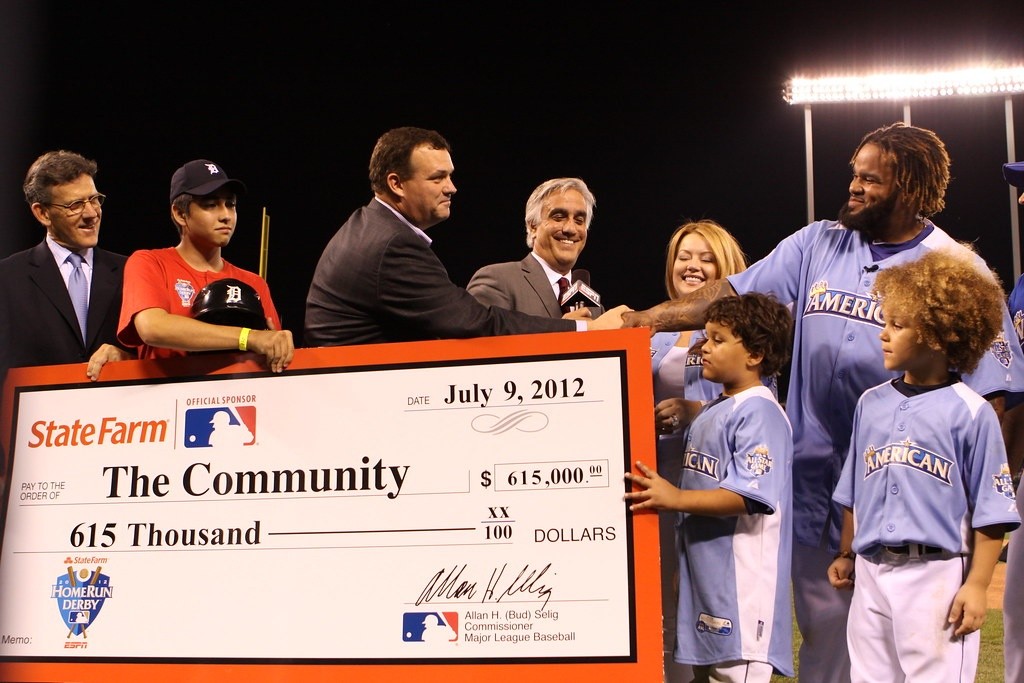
[170,158,240,204]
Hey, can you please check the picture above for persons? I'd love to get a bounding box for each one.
[988,162,1024,682]
[623,119,1024,683]
[621,286,797,683]
[304,126,635,347]
[116,157,294,375]
[824,249,1024,683]
[466,176,607,323]
[649,220,780,682]
[2,149,140,382]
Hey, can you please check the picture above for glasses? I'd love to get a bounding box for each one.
[41,192,106,215]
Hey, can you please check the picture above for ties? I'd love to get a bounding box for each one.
[556,277,570,307]
[67,253,88,347]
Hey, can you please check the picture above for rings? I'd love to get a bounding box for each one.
[671,414,680,429]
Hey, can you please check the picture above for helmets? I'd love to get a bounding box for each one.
[192,278,267,331]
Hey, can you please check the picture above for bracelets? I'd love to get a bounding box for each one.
[238,326,251,352]
[835,550,856,562]
